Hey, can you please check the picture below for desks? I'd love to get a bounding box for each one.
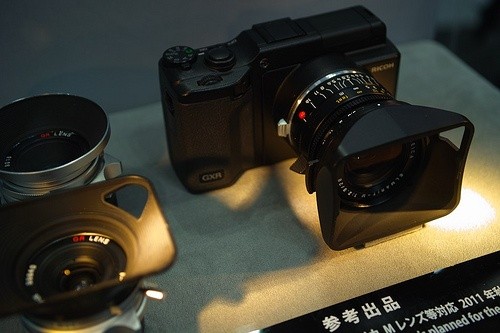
[0,42,500,333]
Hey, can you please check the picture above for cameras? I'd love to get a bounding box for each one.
[159,5,475,251]
